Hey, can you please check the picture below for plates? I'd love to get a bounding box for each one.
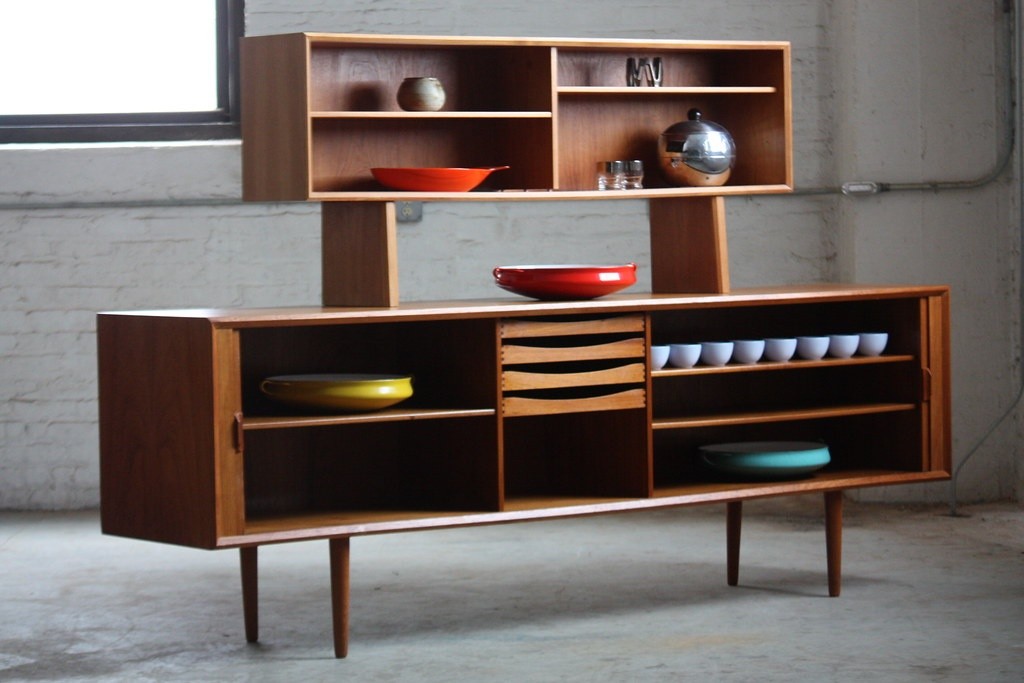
[701,443,832,481]
[494,263,637,300]
[370,165,509,193]
[261,373,414,412]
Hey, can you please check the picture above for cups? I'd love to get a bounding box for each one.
[624,160,643,189]
[597,161,624,190]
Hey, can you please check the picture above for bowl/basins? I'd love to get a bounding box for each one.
[668,344,702,368]
[765,338,797,364]
[652,346,671,370]
[798,337,830,358]
[858,333,888,356]
[701,342,737,364]
[828,334,861,357]
[731,339,766,364]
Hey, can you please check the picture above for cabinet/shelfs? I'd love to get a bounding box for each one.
[237,31,794,204]
[95,284,957,660]
